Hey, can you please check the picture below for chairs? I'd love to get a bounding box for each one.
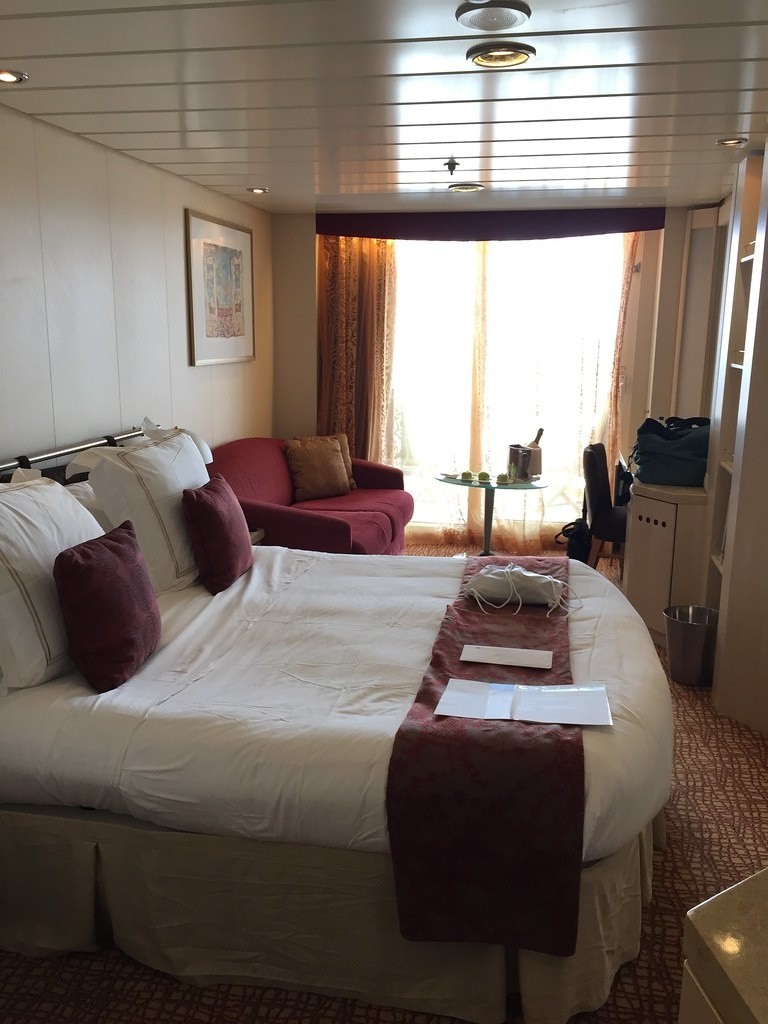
[578,440,625,581]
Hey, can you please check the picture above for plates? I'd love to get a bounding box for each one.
[440,471,460,478]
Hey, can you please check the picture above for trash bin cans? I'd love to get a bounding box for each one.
[663,603,718,686]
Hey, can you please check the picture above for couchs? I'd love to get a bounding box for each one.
[210,429,416,555]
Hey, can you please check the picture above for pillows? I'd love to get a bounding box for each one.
[174,472,256,595]
[0,467,108,692]
[48,519,166,697]
[294,431,356,493]
[69,414,214,594]
[279,437,353,502]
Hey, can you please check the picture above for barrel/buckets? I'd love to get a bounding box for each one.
[508,443,533,480]
[661,603,719,688]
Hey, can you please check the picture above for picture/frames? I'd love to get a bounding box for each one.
[183,206,258,369]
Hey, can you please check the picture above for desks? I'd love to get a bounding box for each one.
[432,473,547,557]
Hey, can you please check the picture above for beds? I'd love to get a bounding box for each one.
[0,417,681,1024]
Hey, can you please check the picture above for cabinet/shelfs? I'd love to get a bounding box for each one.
[624,460,708,651]
[680,867,768,1024]
[705,143,768,750]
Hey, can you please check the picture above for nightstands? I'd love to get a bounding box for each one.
[248,525,266,546]
